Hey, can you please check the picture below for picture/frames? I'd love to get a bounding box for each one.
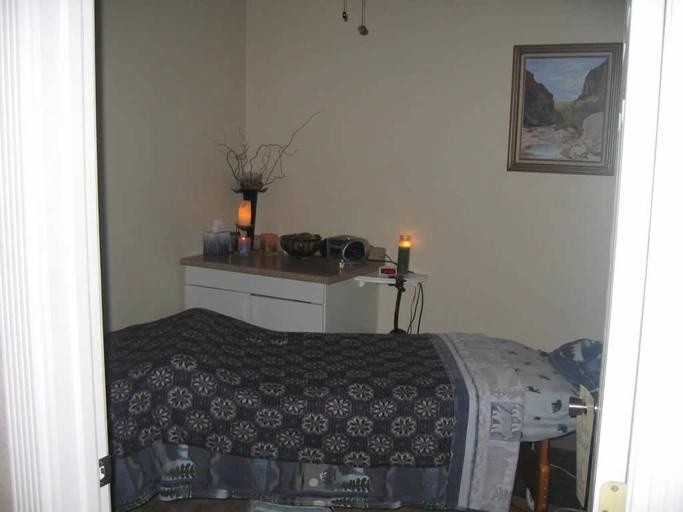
[507,43,624,176]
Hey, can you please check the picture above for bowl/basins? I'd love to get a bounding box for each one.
[280,234,320,255]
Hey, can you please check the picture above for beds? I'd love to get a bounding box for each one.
[101,307,588,511]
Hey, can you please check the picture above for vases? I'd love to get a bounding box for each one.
[234,186,265,247]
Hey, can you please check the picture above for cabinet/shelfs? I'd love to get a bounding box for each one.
[183,266,378,335]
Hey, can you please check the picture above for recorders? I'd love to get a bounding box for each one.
[320,235,370,261]
